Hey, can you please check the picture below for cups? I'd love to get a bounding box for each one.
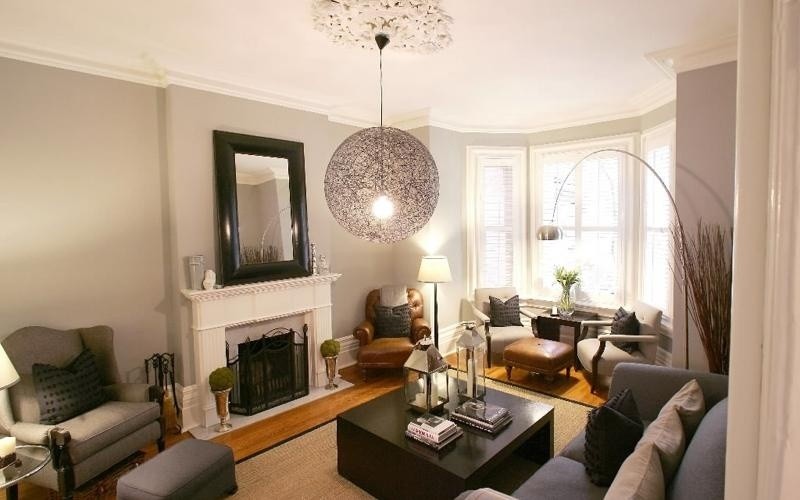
[552,307,557,314]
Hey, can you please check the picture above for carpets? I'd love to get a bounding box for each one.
[221,366,597,499]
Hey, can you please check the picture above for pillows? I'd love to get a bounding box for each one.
[586,380,704,500]
[32,348,108,425]
[610,306,640,354]
[372,301,412,339]
[487,294,524,327]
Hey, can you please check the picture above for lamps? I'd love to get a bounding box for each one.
[0,343,22,467]
[417,256,451,356]
[312,0,457,244]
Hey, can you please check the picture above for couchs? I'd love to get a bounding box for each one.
[451,363,728,500]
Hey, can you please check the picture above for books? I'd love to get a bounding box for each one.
[451,398,514,436]
[405,413,464,450]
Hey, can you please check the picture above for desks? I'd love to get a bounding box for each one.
[537,307,598,369]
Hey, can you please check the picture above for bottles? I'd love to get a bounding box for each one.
[189,255,205,290]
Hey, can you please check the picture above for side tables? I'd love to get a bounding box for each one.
[0,444,51,500]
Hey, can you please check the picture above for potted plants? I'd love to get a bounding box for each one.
[320,339,340,389]
[209,368,234,432]
[551,265,582,317]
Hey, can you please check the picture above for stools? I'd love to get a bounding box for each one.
[116,439,237,500]
[502,335,576,392]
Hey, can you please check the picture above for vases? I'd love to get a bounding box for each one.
[536,148,694,369]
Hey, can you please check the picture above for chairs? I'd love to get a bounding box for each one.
[353,284,433,382]
[0,325,165,498]
[573,299,663,393]
[472,286,538,369]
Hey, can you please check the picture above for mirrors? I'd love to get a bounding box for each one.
[212,130,312,286]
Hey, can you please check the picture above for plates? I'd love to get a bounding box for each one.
[550,314,559,317]
